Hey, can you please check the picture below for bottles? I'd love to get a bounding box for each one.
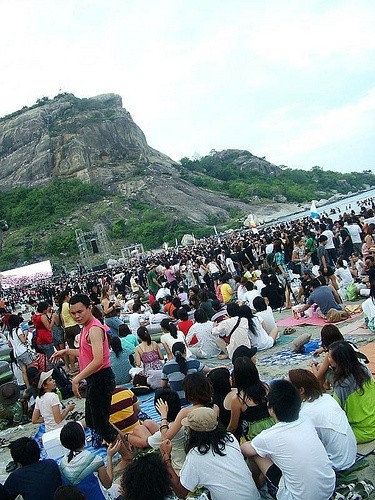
[56,389,62,400]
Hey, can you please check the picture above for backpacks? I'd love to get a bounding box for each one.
[50,366,73,399]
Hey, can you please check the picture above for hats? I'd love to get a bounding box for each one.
[0,383,20,404]
[38,369,55,389]
[232,345,258,365]
[180,407,218,432]
[149,263,157,269]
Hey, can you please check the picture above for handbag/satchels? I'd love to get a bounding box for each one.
[290,333,319,355]
[338,283,359,301]
[293,265,301,275]
[345,304,362,314]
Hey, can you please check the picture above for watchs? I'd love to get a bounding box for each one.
[123,432,132,442]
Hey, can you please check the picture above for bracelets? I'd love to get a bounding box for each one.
[159,424,169,432]
[52,320,55,323]
[112,305,115,311]
[63,410,69,414]
[324,266,327,268]
[0,191,375,500]
[160,418,167,422]
[311,361,320,367]
[282,272,285,273]
[163,458,171,463]
